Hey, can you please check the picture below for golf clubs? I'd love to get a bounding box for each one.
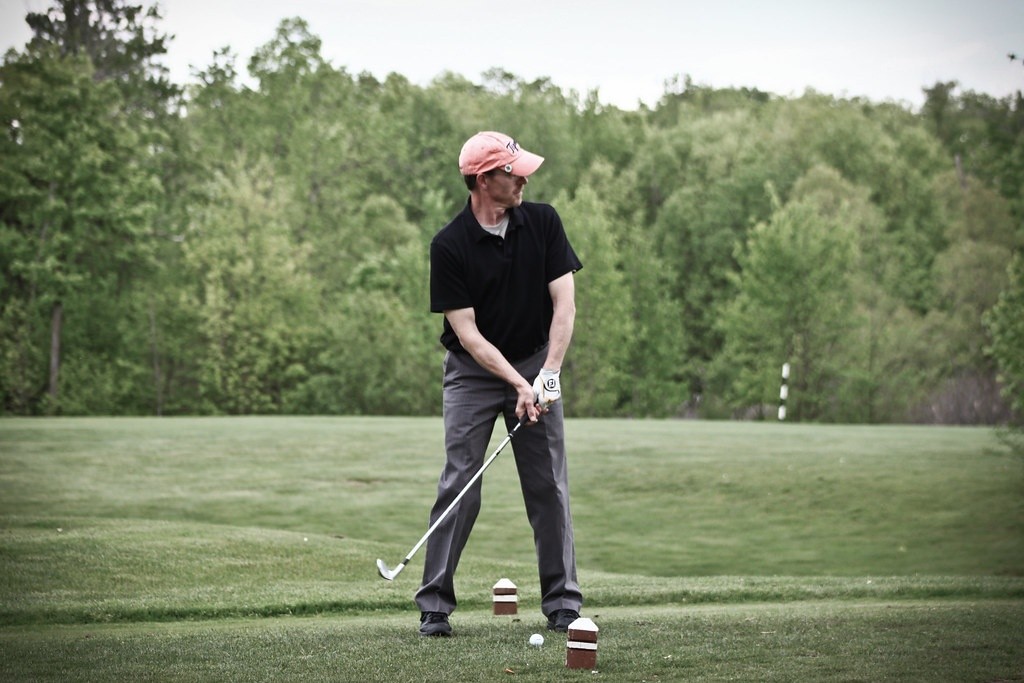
[373,400,539,583]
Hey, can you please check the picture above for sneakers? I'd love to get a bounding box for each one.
[419,611,452,637]
[546,608,580,632]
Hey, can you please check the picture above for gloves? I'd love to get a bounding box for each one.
[529,369,563,413]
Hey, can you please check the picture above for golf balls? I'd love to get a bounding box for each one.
[529,633,544,647]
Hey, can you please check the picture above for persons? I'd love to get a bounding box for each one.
[414,132,583,635]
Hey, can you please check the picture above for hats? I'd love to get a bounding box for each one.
[458,131,546,178]
